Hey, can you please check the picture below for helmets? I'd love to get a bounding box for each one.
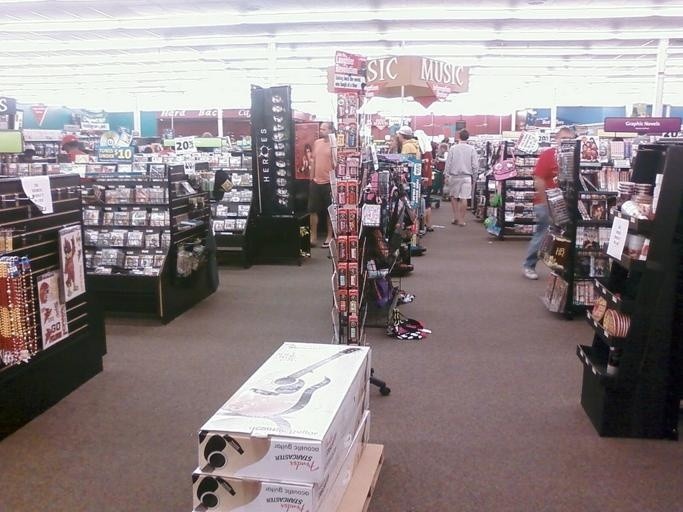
[61,135,79,150]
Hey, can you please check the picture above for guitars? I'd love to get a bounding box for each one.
[223,347,363,418]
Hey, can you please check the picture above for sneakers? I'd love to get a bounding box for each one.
[524,269,538,280]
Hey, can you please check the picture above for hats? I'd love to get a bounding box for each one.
[396,126,412,135]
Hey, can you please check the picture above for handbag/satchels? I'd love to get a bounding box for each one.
[492,160,517,180]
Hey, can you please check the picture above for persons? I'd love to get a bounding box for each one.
[384,126,479,233]
[306,120,336,248]
[61,135,94,162]
[522,127,576,280]
[298,145,313,175]
[356,60,368,92]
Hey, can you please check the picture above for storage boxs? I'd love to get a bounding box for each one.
[191,341,371,512]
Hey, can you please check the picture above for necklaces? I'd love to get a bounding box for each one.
[0,255,39,367]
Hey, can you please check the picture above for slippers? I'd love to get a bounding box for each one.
[321,242,330,248]
[451,218,466,227]
[426,227,434,232]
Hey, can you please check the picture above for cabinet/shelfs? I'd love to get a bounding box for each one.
[330,132,444,396]
[201,132,315,270]
[555,132,682,438]
[1,132,107,437]
[81,130,220,326]
[468,132,544,241]
